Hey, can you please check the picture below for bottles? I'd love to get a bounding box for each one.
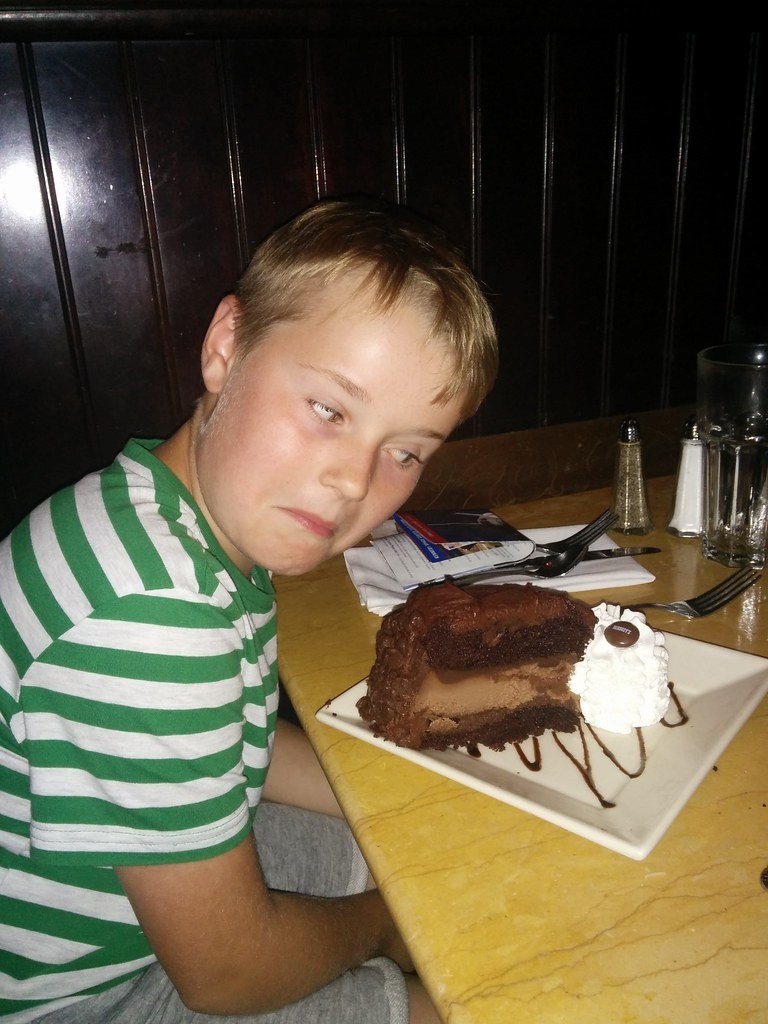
[608,419,655,537]
[667,419,709,539]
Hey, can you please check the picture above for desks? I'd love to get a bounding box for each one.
[275,468,767,1023]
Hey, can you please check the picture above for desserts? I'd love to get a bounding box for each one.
[354,575,670,752]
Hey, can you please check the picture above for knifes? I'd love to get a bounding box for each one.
[506,546,663,569]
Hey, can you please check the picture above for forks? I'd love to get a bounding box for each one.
[533,506,621,556]
[602,566,764,619]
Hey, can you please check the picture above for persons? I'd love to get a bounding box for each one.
[0,200,497,1024]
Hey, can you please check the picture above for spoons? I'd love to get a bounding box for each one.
[435,543,589,589]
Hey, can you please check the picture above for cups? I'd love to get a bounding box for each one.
[699,337,768,568]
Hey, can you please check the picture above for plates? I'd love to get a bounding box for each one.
[315,600,768,859]
